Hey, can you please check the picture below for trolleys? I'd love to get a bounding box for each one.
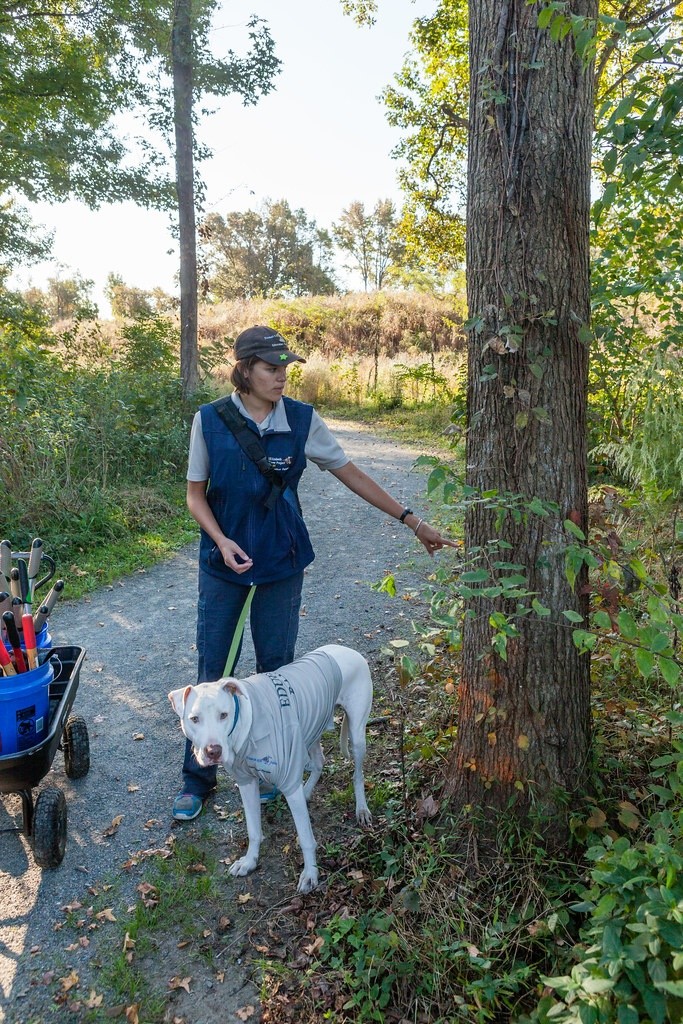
[0,554,91,869]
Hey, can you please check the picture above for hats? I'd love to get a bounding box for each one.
[233,324,307,367]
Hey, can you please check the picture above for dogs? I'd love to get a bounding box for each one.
[167,644,373,894]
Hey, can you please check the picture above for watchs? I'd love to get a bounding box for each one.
[399,507,413,524]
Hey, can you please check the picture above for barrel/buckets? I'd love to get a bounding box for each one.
[0,656,54,756]
[0,620,52,653]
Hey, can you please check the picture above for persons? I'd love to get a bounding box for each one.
[171,325,460,821]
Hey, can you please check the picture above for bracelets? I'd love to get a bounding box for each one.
[414,519,423,533]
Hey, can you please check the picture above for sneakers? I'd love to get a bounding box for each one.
[258,778,280,804]
[171,787,204,820]
